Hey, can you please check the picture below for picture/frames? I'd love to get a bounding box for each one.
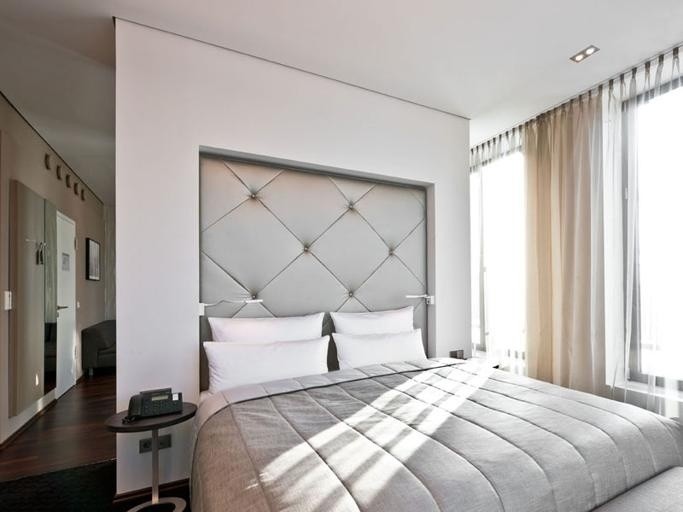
[85,237,101,281]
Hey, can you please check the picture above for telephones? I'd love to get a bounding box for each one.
[128,389,183,420]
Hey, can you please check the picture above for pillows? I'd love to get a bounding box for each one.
[331,327,427,372]
[198,334,332,409]
[207,311,326,342]
[329,305,416,334]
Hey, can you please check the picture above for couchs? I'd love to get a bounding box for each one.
[81,320,116,377]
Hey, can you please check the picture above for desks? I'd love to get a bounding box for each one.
[104,402,197,512]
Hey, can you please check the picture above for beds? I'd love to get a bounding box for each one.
[186,356,683,512]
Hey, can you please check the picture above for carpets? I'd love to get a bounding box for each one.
[1,458,192,512]
